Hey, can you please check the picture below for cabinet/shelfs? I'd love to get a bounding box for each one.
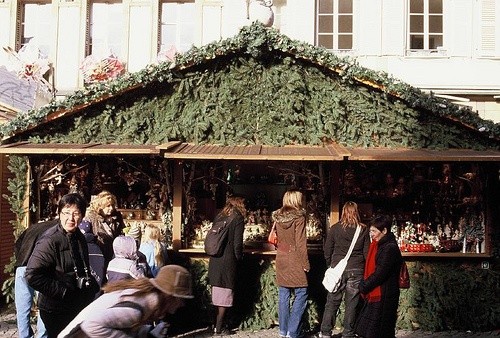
[116,209,165,229]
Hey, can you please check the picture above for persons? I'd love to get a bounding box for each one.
[268,190,311,338]
[320,201,370,338]
[208,196,246,335]
[353,215,402,338]
[13,191,194,338]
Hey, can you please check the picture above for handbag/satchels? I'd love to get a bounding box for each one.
[321,225,362,293]
[64,300,146,338]
[267,221,278,247]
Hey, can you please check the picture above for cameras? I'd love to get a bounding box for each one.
[77,277,92,289]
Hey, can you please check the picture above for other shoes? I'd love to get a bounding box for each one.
[207,326,235,336]
[314,331,362,338]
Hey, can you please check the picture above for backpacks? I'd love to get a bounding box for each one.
[204,212,239,255]
[399,263,410,289]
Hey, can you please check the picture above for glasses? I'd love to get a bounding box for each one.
[60,210,81,216]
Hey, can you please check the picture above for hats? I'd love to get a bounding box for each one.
[150,265,193,299]
